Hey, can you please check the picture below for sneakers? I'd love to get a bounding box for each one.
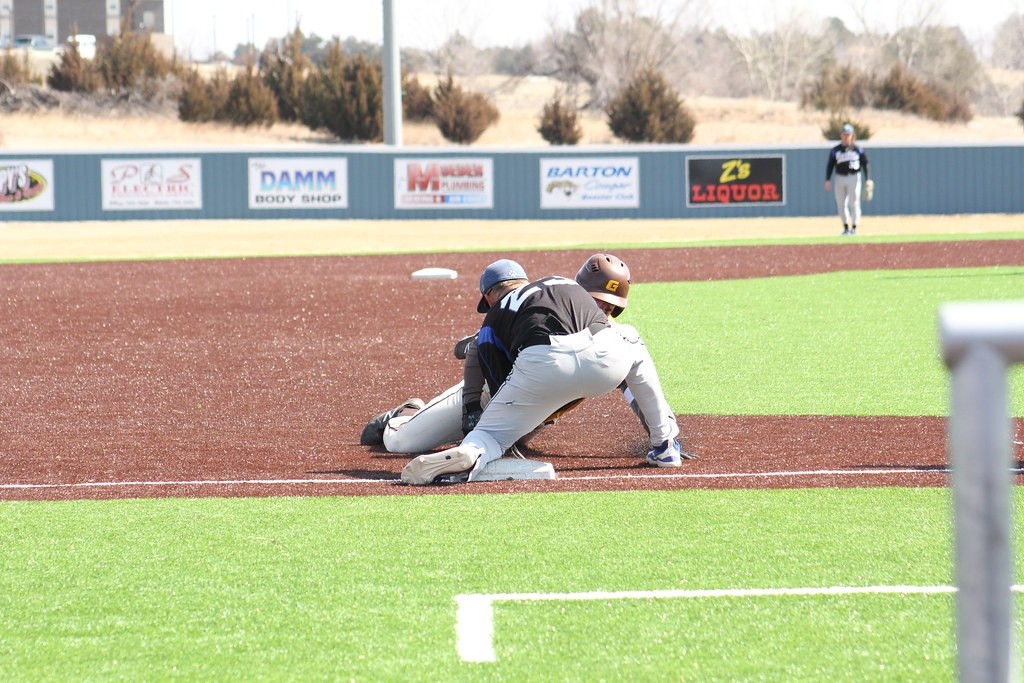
[360,398,426,445]
[843,228,857,236]
[454,328,481,359]
[401,443,485,484]
[646,439,681,467]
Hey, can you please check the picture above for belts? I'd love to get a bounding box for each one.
[836,170,861,177]
[518,323,609,350]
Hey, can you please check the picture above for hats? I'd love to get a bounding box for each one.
[575,253,631,318]
[841,124,854,134]
[477,259,528,314]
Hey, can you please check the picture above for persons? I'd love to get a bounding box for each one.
[824,123,869,234]
[401,258,681,483]
[361,253,631,452]
